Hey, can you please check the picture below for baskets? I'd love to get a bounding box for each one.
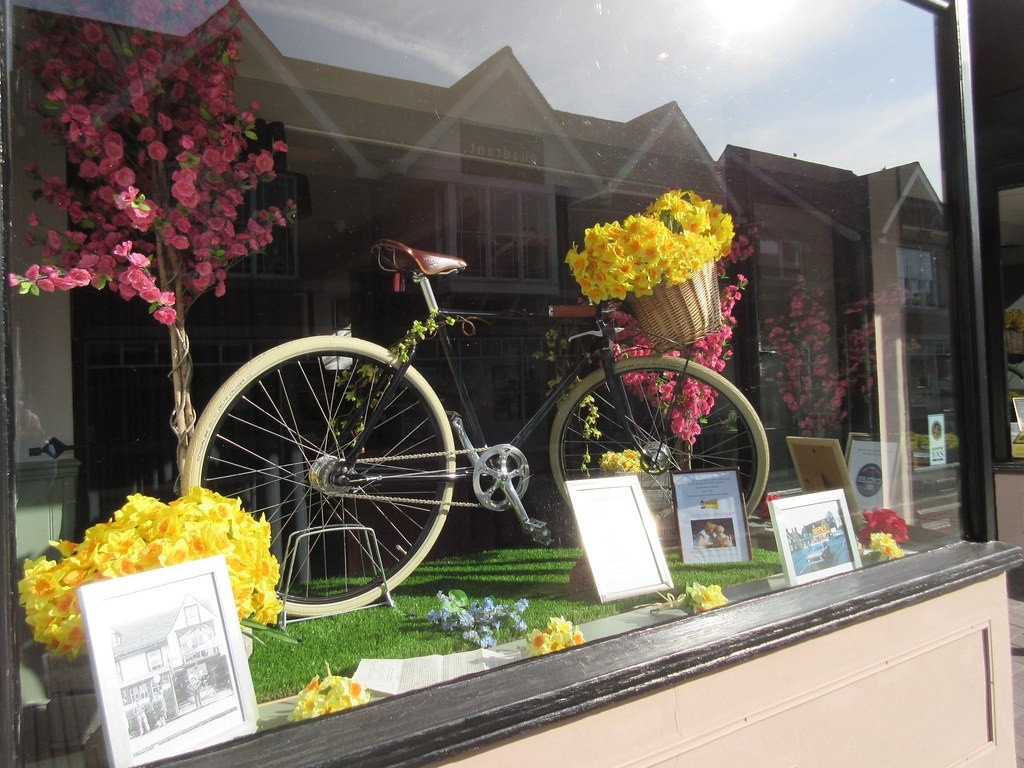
[626,260,723,354]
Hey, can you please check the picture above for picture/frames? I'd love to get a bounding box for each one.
[669,465,753,564]
[76,554,261,768]
[785,435,864,523]
[564,475,674,604]
[767,487,863,588]
[844,432,910,513]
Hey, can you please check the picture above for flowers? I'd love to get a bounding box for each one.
[0,1,911,726]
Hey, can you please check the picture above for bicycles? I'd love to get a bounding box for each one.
[173,224,774,632]
